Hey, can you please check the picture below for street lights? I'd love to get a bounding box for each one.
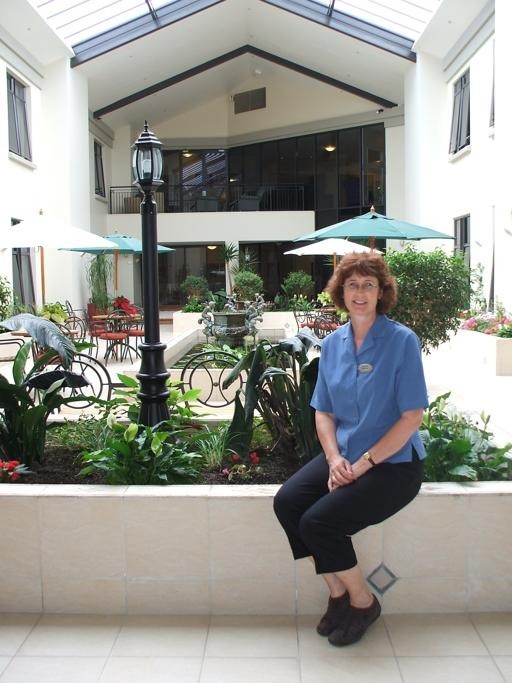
[130,119,171,432]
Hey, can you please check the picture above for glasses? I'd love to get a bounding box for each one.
[342,281,377,291]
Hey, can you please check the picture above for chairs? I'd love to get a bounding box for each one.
[293,302,344,339]
[0,300,145,367]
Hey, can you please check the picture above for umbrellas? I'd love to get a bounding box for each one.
[291,205,457,257]
[282,237,385,273]
[57,229,177,298]
[0,208,119,306]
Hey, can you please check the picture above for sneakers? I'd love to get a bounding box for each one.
[317,589,381,645]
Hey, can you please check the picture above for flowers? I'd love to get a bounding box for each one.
[111,295,135,312]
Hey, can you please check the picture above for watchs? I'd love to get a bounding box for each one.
[363,452,376,466]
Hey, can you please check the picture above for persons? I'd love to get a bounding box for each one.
[273,251,431,647]
